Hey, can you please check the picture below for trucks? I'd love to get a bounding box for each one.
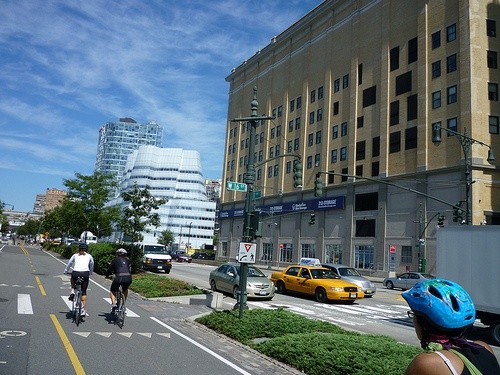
[436,225,500,347]
[80,232,97,244]
[66,239,75,245]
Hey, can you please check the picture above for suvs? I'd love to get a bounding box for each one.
[138,243,172,274]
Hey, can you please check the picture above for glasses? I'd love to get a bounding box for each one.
[407,310,418,319]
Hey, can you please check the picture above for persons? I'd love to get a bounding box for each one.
[39,237,45,249]
[63,243,94,317]
[400,278,500,375]
[105,248,132,316]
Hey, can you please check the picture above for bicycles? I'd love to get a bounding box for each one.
[64,273,91,326]
[104,276,126,328]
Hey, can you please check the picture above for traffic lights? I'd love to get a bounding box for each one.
[310,214,315,224]
[438,216,445,228]
[452,202,463,222]
[293,163,302,188]
[314,176,323,197]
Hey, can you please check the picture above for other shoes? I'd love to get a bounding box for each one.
[111,304,117,315]
[82,310,85,316]
[69,292,75,302]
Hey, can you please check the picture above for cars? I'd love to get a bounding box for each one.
[192,252,214,260]
[383,272,435,290]
[270,258,364,304]
[321,263,376,296]
[209,262,275,301]
[172,250,192,263]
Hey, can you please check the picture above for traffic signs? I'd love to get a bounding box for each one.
[251,190,262,199]
[226,181,247,192]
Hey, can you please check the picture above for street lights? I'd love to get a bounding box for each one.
[431,124,495,226]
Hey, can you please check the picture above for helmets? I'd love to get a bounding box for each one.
[79,243,89,251]
[116,248,127,254]
[401,277,476,328]
